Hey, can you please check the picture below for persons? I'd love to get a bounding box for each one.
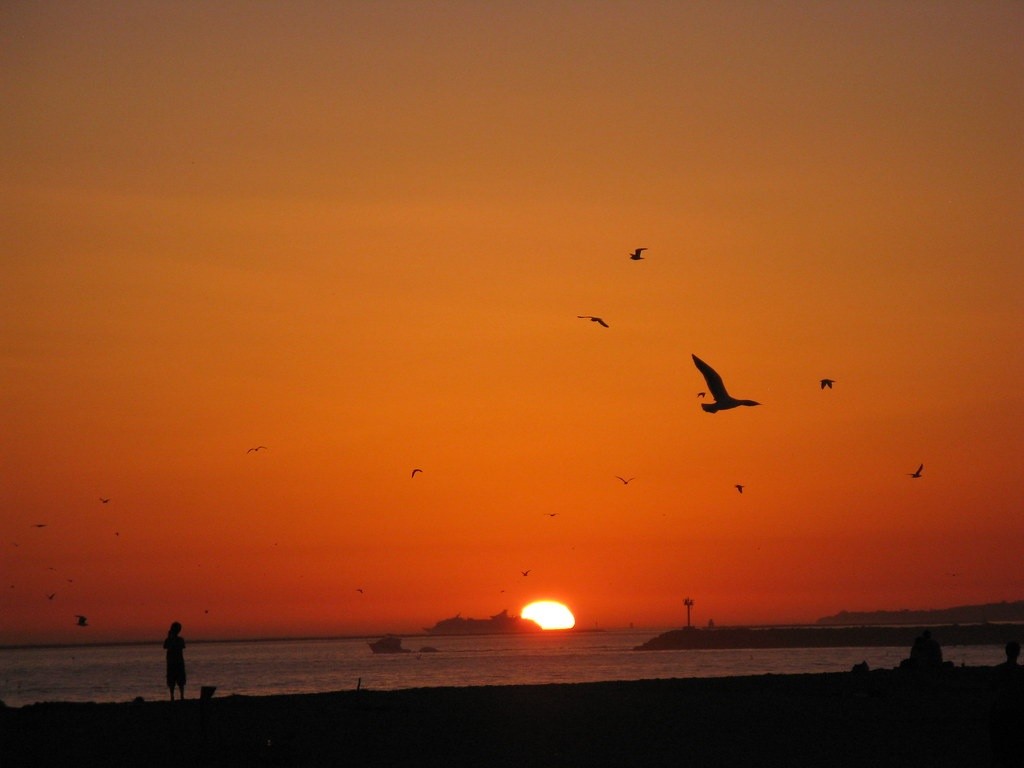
[163,621,187,700]
[901,631,1024,768]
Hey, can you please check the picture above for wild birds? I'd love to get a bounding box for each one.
[34,524,47,528]
[246,446,267,454]
[520,569,532,576]
[47,593,55,599]
[820,378,836,390]
[100,497,109,503]
[615,475,635,485]
[735,484,744,494]
[904,463,924,478]
[13,542,22,547]
[411,468,423,477]
[691,353,762,414]
[577,315,610,328]
[356,589,363,594]
[543,513,560,517]
[629,247,648,260]
[74,615,89,628]
